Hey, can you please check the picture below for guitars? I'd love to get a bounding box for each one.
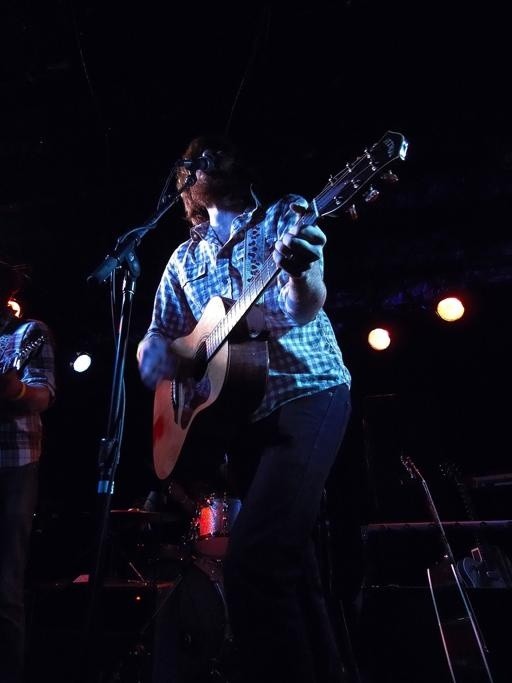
[437,465,505,588]
[400,456,496,683]
[153,130,409,478]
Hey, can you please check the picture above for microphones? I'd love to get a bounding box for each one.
[176,150,219,176]
[400,453,427,484]
[440,462,462,484]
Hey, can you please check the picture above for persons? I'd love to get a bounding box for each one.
[0,262,60,683]
[133,132,357,682]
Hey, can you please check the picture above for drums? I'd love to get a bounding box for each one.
[190,497,246,556]
